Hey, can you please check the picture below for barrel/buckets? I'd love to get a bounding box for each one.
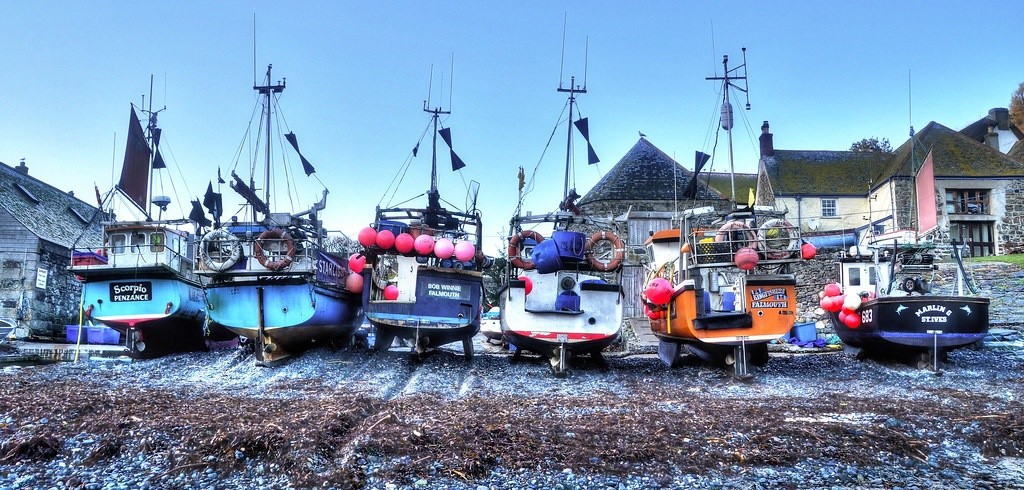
[552,231,586,260]
[530,239,561,274]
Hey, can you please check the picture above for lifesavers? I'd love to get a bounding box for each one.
[256,228,295,271]
[200,230,243,272]
[714,222,758,251]
[757,220,799,259]
[508,231,544,269]
[585,232,624,272]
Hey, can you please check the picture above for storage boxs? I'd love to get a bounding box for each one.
[793,321,817,344]
[88,327,120,343]
[65,325,87,342]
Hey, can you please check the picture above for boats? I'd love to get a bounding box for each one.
[480,307,503,342]
[188,13,364,363]
[496,13,634,378]
[828,70,991,373]
[361,52,495,360]
[65,73,237,358]
[639,19,816,376]
